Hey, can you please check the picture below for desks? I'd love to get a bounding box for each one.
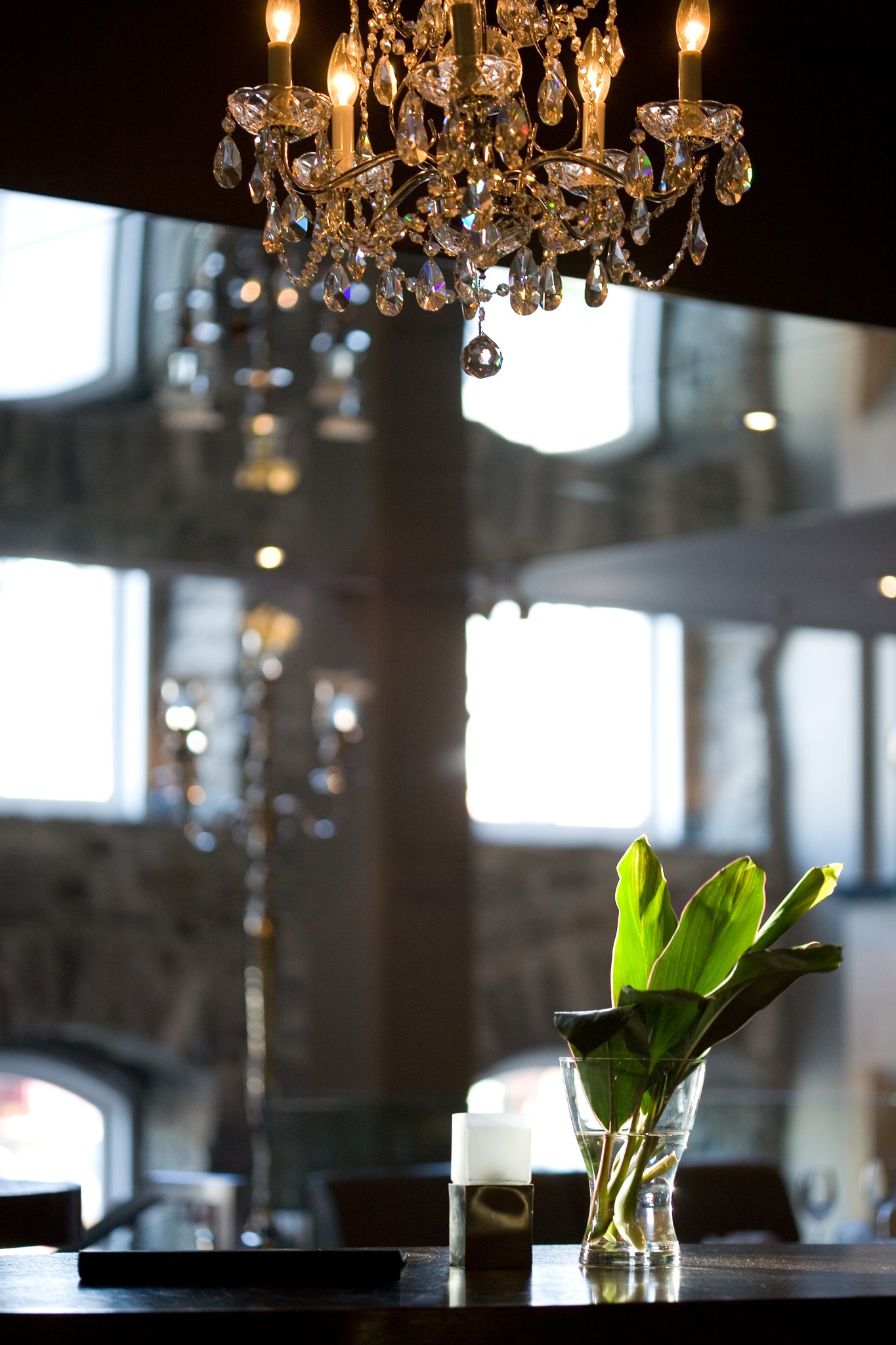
[0,1184,895,1345]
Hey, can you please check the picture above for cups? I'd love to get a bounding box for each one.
[241,1210,312,1248]
[137,1172,234,1249]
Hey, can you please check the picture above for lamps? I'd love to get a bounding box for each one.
[210,0,755,380]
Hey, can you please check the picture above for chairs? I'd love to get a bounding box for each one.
[537,1162,803,1248]
[0,1184,88,1255]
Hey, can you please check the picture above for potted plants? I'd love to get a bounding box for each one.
[547,838,848,1276]
